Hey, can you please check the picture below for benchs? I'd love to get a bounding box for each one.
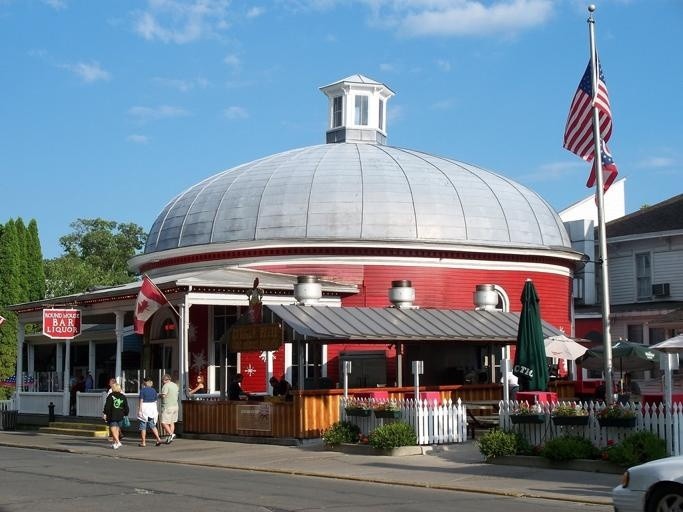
[465,404,492,439]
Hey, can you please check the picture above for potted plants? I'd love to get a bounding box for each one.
[475,428,670,472]
[319,421,422,456]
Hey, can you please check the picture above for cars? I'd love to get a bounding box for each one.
[611,453,683,512]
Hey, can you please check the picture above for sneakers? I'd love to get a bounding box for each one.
[111,440,123,450]
[155,440,162,445]
[164,433,176,444]
[138,443,147,447]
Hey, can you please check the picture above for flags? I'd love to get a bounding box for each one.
[0,316,6,326]
[561,51,619,206]
[132,279,168,334]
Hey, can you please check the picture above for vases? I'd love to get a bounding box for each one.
[509,414,636,428]
[346,409,395,417]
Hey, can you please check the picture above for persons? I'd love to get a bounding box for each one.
[188,375,206,395]
[103,377,125,440]
[246,277,264,324]
[102,383,129,450]
[159,374,180,444]
[617,373,641,395]
[136,376,162,447]
[269,376,293,402]
[228,373,250,401]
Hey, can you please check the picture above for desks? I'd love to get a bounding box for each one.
[466,401,499,426]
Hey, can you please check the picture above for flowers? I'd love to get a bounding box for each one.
[346,398,397,413]
[512,400,636,417]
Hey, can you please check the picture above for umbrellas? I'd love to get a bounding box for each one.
[512,277,551,391]
[575,336,660,395]
[1,374,37,386]
[544,334,590,361]
